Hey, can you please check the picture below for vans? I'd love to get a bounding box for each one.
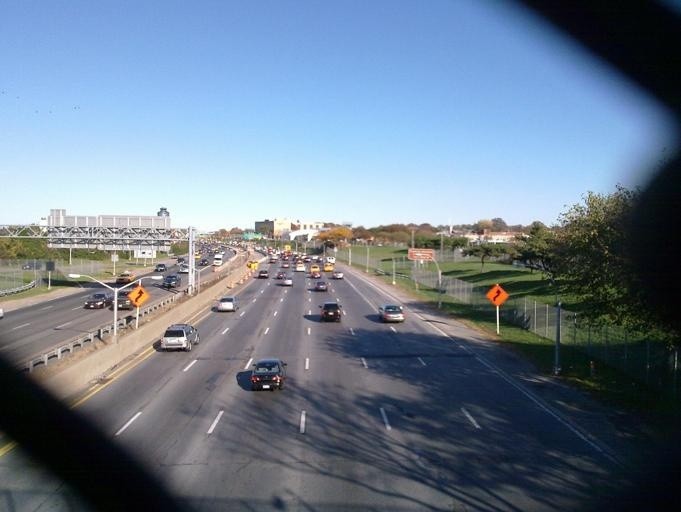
[159,321,201,353]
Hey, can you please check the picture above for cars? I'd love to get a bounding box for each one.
[21,260,41,270]
[247,358,290,392]
[316,299,343,323]
[114,270,135,284]
[152,241,344,291]
[109,288,136,310]
[376,303,408,323]
[80,288,112,310]
[216,293,241,314]
[0,305,5,320]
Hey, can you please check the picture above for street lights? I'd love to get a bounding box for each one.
[67,270,166,338]
[415,251,443,311]
[523,262,564,376]
[177,261,216,295]
[339,238,398,285]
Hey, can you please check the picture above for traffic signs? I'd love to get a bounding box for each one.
[45,261,54,271]
[407,248,435,262]
